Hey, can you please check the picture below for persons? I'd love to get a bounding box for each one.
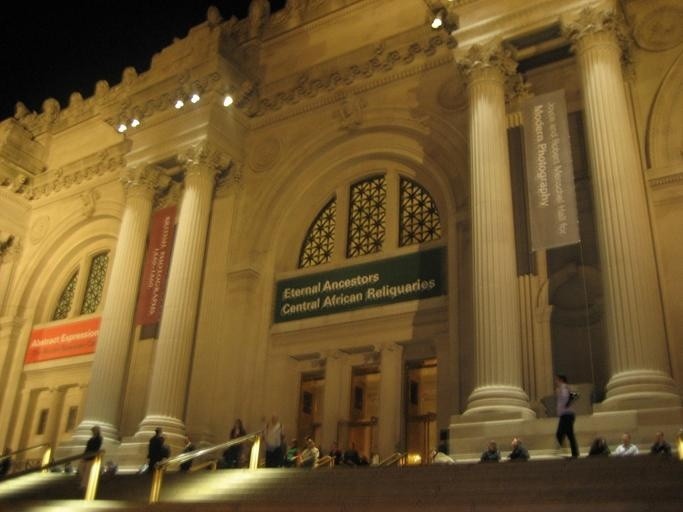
[587,436,610,456]
[477,441,501,460]
[105,460,119,477]
[260,414,371,468]
[0,448,13,481]
[179,436,195,470]
[227,417,247,468]
[647,431,672,453]
[553,375,579,457]
[507,438,531,461]
[76,424,102,476]
[608,433,639,456]
[156,434,169,468]
[146,427,163,470]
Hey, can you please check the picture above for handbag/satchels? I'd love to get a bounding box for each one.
[565,392,578,407]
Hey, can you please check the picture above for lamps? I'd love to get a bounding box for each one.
[119,108,144,133]
[429,7,449,30]
[171,81,236,110]
